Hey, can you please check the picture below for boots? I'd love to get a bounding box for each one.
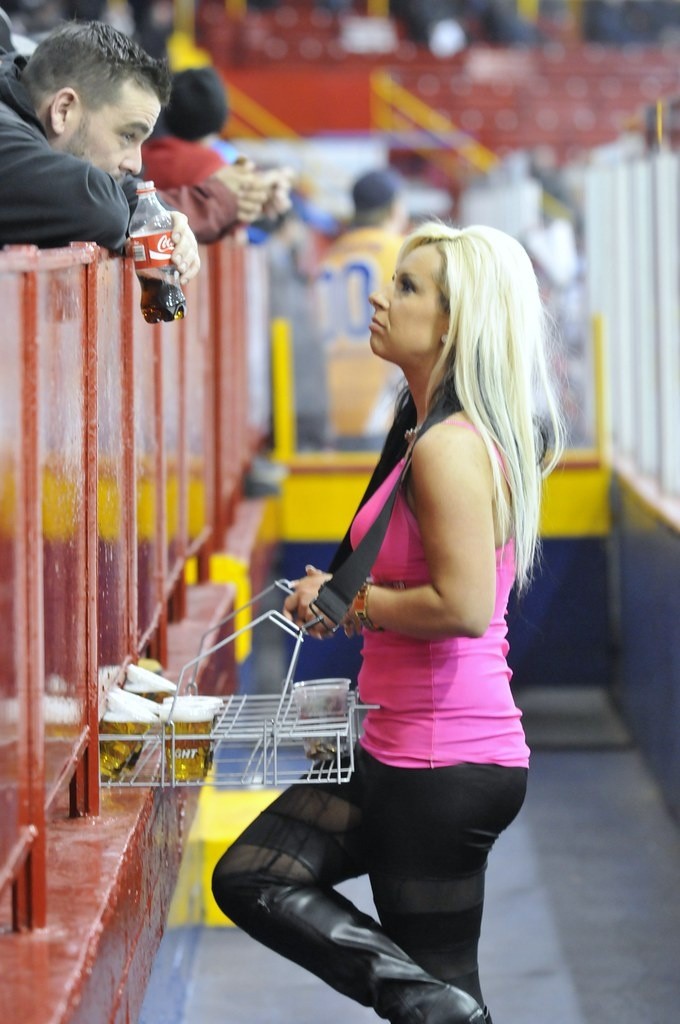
[211,871,485,1024]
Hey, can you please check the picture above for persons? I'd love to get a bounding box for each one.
[309,169,412,455]
[1,0,315,270]
[209,223,569,1024]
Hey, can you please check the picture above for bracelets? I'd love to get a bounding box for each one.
[344,583,384,639]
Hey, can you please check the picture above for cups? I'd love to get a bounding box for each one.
[100,663,223,782]
[294,677,352,759]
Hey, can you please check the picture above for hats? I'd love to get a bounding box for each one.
[166,68,229,139]
[353,173,400,206]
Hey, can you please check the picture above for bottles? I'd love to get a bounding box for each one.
[129,180,187,324]
